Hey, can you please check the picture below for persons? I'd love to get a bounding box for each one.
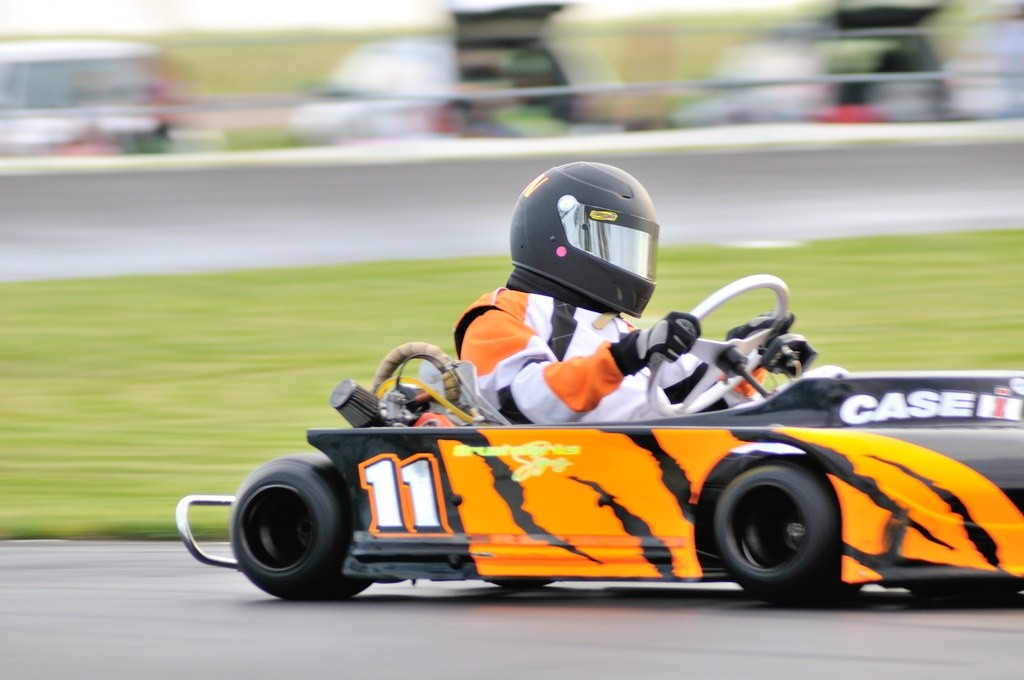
[453,160,796,423]
[450,0,577,126]
[832,0,950,106]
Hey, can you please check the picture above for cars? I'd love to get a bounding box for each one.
[667,3,951,128]
[1,38,172,159]
[318,2,579,141]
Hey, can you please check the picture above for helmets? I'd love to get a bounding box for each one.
[507,162,658,317]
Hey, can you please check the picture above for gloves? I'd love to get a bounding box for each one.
[610,311,702,376]
[728,312,796,353]
